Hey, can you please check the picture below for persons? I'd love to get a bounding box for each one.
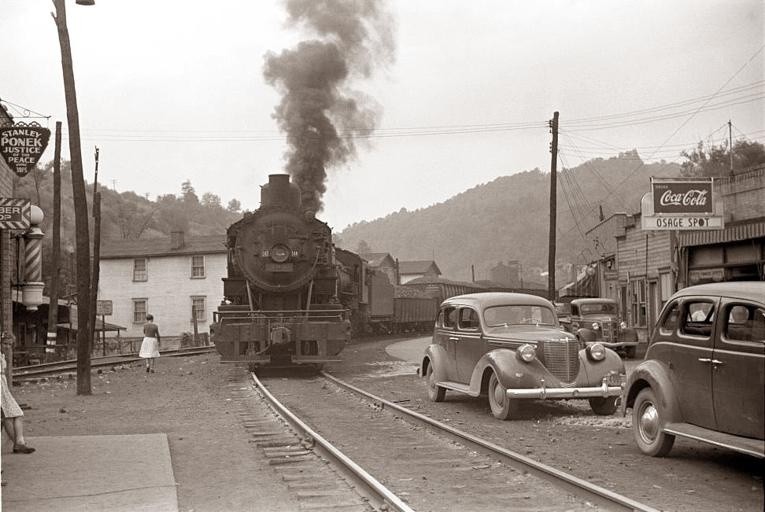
[686,302,715,322]
[139,313,162,374]
[0,331,37,455]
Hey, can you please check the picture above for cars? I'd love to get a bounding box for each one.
[557,297,641,359]
[413,289,629,421]
[612,278,764,463]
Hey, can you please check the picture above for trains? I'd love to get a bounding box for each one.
[208,173,559,378]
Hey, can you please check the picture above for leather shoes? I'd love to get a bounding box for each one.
[14,445,35,453]
